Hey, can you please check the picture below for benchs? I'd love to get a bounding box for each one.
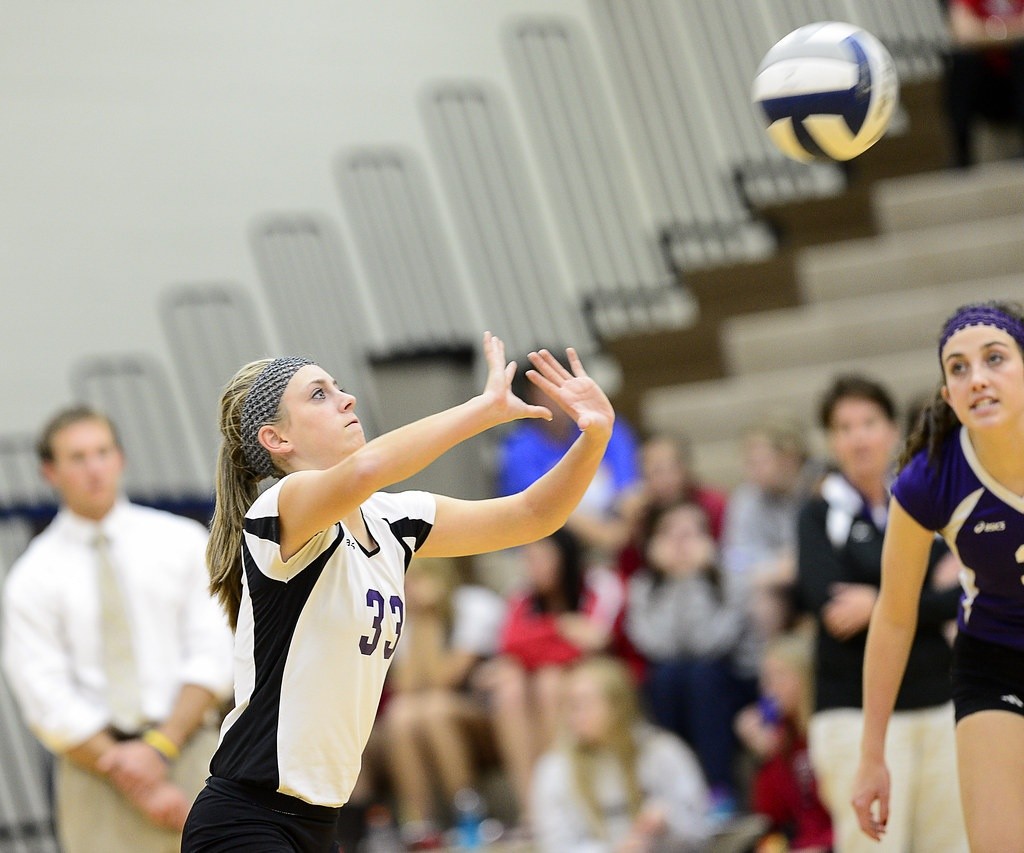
[589,78,1024,489]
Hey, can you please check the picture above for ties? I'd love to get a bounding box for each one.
[94,536,142,735]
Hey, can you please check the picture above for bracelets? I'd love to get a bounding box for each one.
[140,729,177,761]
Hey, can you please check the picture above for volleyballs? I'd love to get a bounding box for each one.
[749,18,902,169]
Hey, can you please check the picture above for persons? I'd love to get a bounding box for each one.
[854,297,1022,853]
[350,350,830,853]
[4,407,239,852]
[180,330,615,852]
[796,374,969,853]
[937,1,1024,165]
